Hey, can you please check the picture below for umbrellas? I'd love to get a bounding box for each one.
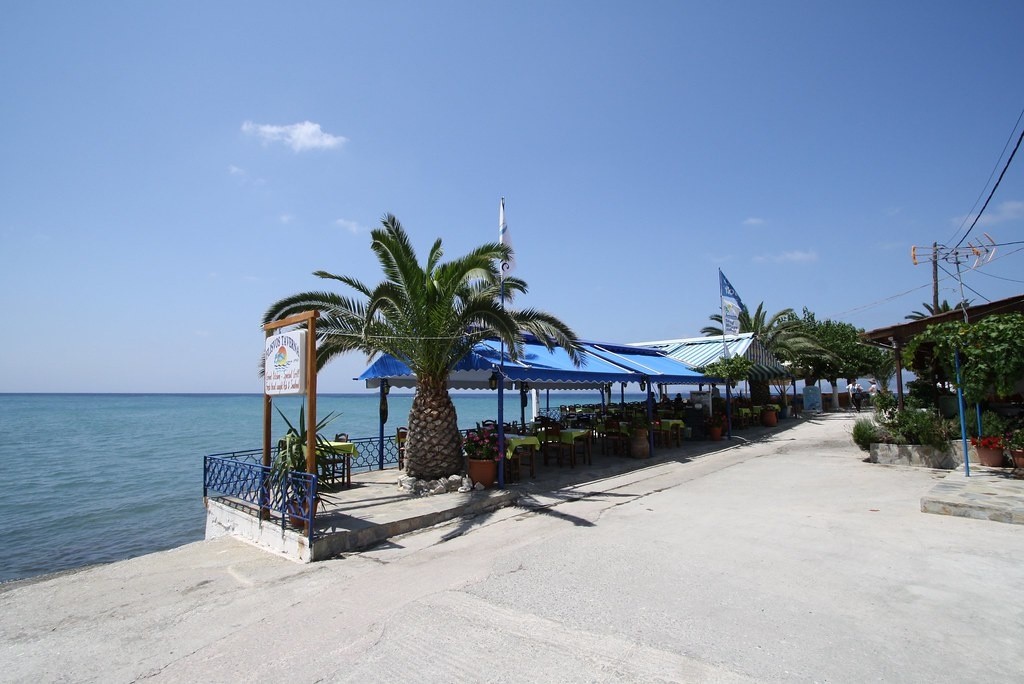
[767,359,813,407]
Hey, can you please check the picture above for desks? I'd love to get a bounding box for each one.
[559,407,573,416]
[595,421,631,456]
[529,421,551,435]
[562,419,579,428]
[316,442,359,486]
[608,408,621,415]
[574,408,593,413]
[750,406,763,426]
[493,433,540,482]
[657,410,682,420]
[537,428,592,469]
[733,408,752,429]
[766,404,781,423]
[644,419,685,450]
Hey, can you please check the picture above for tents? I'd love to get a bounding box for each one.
[572,339,740,455]
[625,332,796,439]
[355,325,644,486]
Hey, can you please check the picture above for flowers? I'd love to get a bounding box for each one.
[703,413,727,428]
[1005,427,1024,451]
[771,396,792,407]
[463,426,510,461]
[625,416,660,432]
[762,405,779,412]
[971,434,1008,450]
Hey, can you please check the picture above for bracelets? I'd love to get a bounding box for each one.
[864,391,866,394]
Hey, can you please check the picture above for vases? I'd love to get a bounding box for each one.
[708,427,723,441]
[976,447,1004,466]
[777,406,792,419]
[1010,449,1024,468]
[468,458,498,488]
[628,430,650,459]
[762,411,777,427]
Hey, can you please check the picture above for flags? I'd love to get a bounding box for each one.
[718,268,748,336]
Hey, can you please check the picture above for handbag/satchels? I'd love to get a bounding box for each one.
[850,384,856,392]
[854,389,862,399]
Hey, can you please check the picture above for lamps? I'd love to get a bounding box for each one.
[488,373,498,390]
[624,383,627,388]
[639,382,647,392]
[605,385,608,392]
[524,384,530,392]
[384,381,392,395]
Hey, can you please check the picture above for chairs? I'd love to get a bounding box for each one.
[317,433,349,485]
[397,391,873,483]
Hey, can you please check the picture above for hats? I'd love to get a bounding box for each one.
[868,378,876,383]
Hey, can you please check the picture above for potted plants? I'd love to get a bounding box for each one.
[262,401,352,527]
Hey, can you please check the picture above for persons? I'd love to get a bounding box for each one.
[860,378,877,404]
[846,378,863,414]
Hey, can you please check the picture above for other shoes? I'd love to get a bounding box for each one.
[856,411,860,413]
